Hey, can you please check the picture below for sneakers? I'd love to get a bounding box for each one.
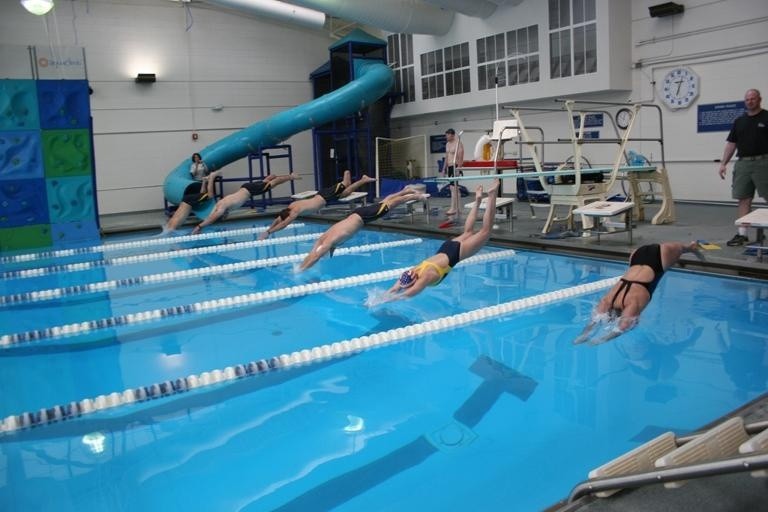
[728,235,749,246]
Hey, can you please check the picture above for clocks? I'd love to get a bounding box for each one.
[659,66,699,109]
[616,109,633,130]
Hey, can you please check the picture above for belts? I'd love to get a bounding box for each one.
[738,154,767,161]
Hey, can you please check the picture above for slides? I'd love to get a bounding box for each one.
[163,57,393,222]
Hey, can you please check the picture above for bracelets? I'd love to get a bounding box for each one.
[267,231,270,234]
[197,224,201,228]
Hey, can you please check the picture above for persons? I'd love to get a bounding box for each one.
[572,241,707,348]
[368,178,500,307]
[441,128,464,215]
[719,89,768,246]
[190,153,209,180]
[167,172,221,230]
[192,171,302,235]
[300,188,427,271]
[258,170,377,241]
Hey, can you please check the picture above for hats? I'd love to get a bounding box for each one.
[316,245,328,258]
[399,269,418,288]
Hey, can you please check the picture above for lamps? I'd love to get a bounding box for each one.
[158,333,186,371]
[344,413,364,467]
[19,0,55,16]
[135,74,156,83]
[647,2,684,18]
[82,431,108,455]
